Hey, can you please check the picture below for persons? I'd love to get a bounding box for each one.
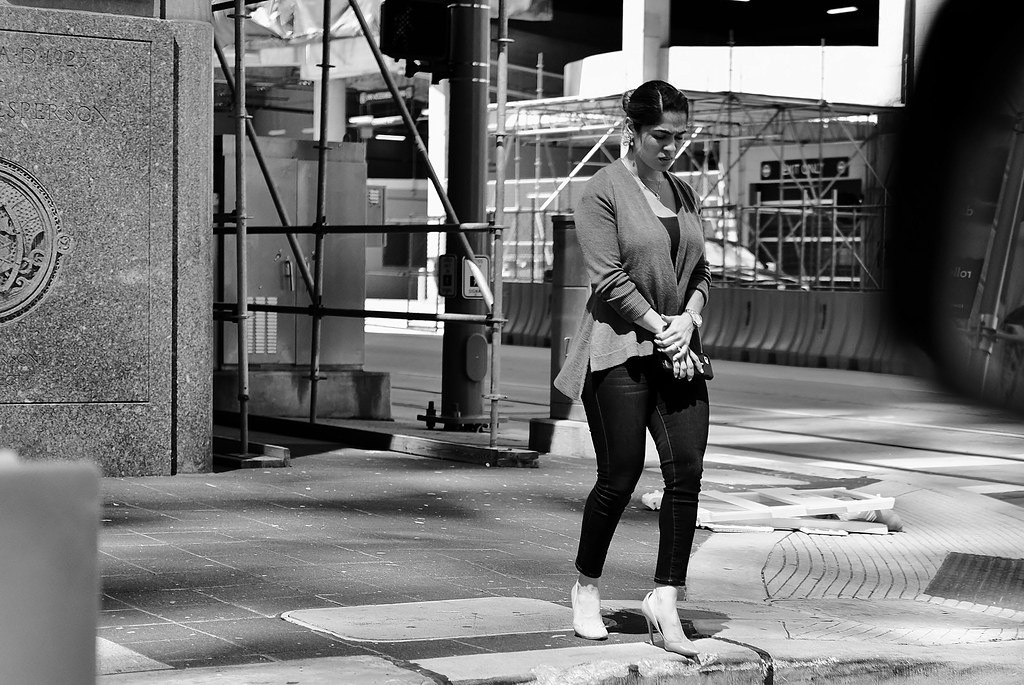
[554,79,712,654]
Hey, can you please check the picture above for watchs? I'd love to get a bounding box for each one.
[685,308,703,329]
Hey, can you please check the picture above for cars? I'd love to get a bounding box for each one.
[705,237,812,291]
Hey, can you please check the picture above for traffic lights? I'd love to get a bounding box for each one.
[379,0,452,62]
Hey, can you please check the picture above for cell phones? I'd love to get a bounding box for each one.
[662,352,714,380]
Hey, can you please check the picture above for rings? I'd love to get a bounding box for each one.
[673,342,682,351]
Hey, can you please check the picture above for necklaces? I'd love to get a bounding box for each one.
[620,157,663,201]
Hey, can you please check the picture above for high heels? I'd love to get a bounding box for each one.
[571,579,608,640]
[641,592,700,656]
